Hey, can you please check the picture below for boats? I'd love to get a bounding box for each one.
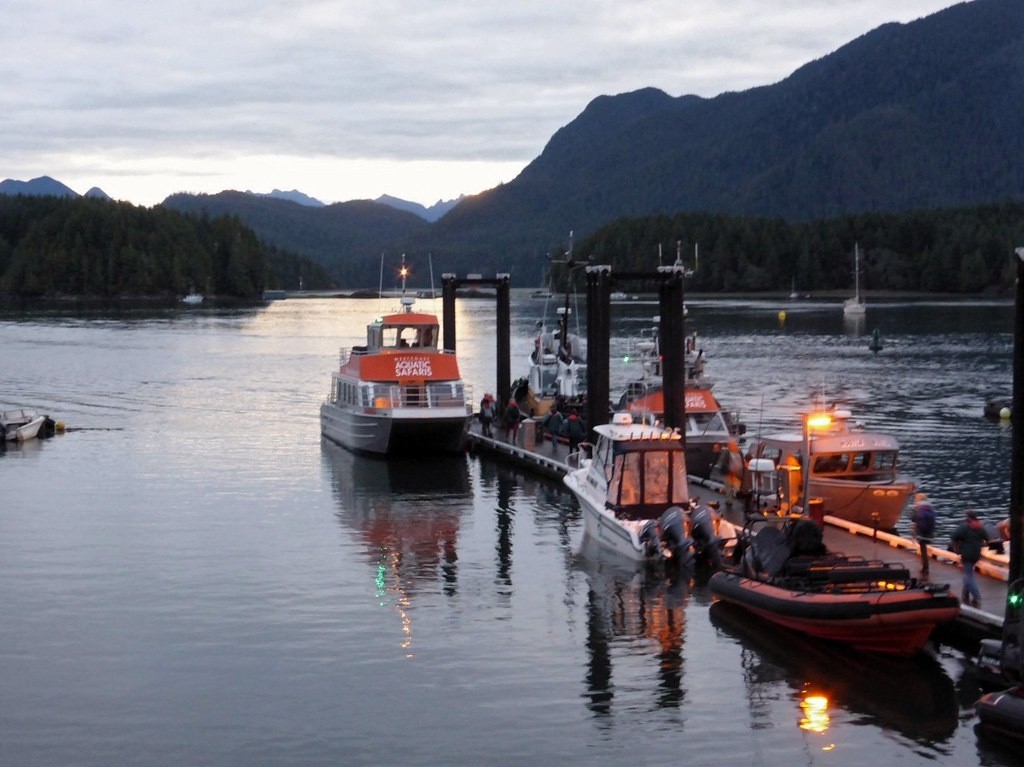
[619,240,749,473]
[868,329,882,351]
[0,407,55,452]
[321,253,473,459]
[710,457,961,655]
[182,287,204,302]
[739,375,915,531]
[531,289,552,298]
[511,276,615,439]
[563,413,737,563]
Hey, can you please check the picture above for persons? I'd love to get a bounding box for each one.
[564,409,585,454]
[480,393,496,439]
[948,510,990,609]
[542,404,564,452]
[910,493,936,577]
[503,398,519,444]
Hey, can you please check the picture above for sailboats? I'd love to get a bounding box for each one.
[843,242,865,314]
[789,274,799,298]
